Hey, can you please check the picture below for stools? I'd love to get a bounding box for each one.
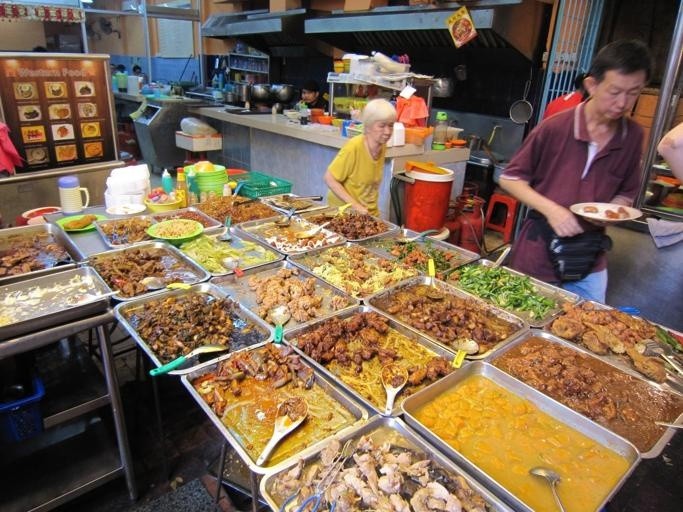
[483,189,521,246]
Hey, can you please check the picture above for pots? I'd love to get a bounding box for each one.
[245,83,295,108]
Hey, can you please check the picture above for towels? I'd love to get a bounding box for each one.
[645,217,682,250]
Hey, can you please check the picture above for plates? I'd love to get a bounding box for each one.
[442,139,467,150]
[21,206,60,226]
[104,203,147,215]
[144,219,203,240]
[53,214,106,232]
[183,164,229,202]
[568,201,644,224]
[15,82,101,163]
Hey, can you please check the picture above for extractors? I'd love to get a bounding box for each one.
[200,0,314,38]
[304,0,508,59]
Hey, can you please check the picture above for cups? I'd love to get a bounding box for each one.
[153,87,160,97]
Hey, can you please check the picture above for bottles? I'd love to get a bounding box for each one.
[162,168,172,193]
[223,184,233,197]
[175,167,188,209]
[431,111,448,152]
[57,175,90,215]
[299,105,308,124]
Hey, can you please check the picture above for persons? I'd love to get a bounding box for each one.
[323,98,398,220]
[493,35,650,307]
[654,120,682,190]
[289,78,334,112]
[540,71,589,119]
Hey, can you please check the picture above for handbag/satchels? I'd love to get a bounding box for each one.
[525,210,614,285]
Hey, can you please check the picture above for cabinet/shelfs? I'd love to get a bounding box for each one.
[327,70,437,135]
[228,50,287,84]
[0,305,141,512]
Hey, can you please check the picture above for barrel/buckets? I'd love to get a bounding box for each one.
[402,166,456,237]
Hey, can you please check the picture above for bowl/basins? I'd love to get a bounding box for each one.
[282,110,298,121]
[333,58,350,74]
[145,194,182,214]
[309,109,344,128]
